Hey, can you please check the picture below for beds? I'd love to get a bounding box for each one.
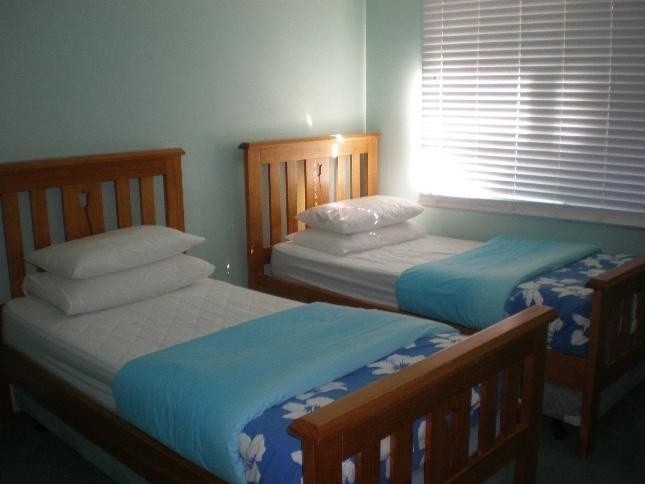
[1,147,559,480]
[238,133,643,484]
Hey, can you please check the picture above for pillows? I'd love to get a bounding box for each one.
[22,223,207,280]
[293,194,425,235]
[22,253,216,316]
[284,220,428,256]
